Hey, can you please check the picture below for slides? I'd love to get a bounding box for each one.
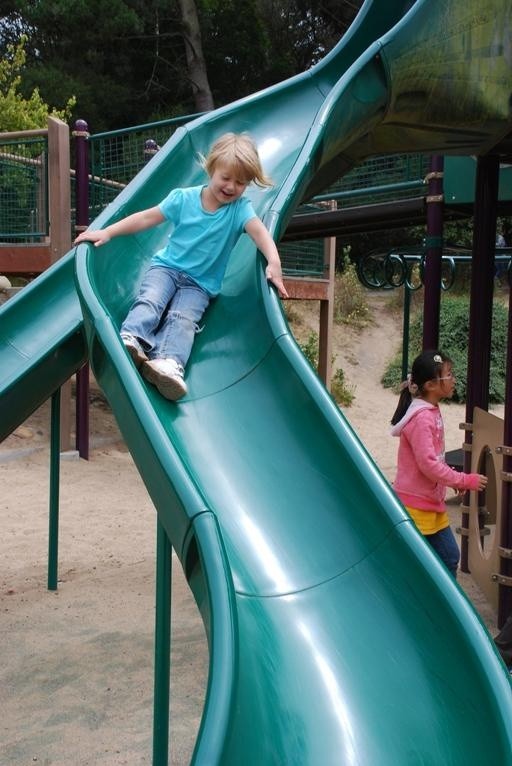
[0,250,88,442]
[76,0,511,765]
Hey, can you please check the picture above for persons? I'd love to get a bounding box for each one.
[390,351,488,580]
[74,131,290,401]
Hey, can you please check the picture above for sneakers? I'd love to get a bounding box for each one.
[120,331,188,401]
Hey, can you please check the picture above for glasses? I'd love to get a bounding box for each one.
[433,373,452,383]
